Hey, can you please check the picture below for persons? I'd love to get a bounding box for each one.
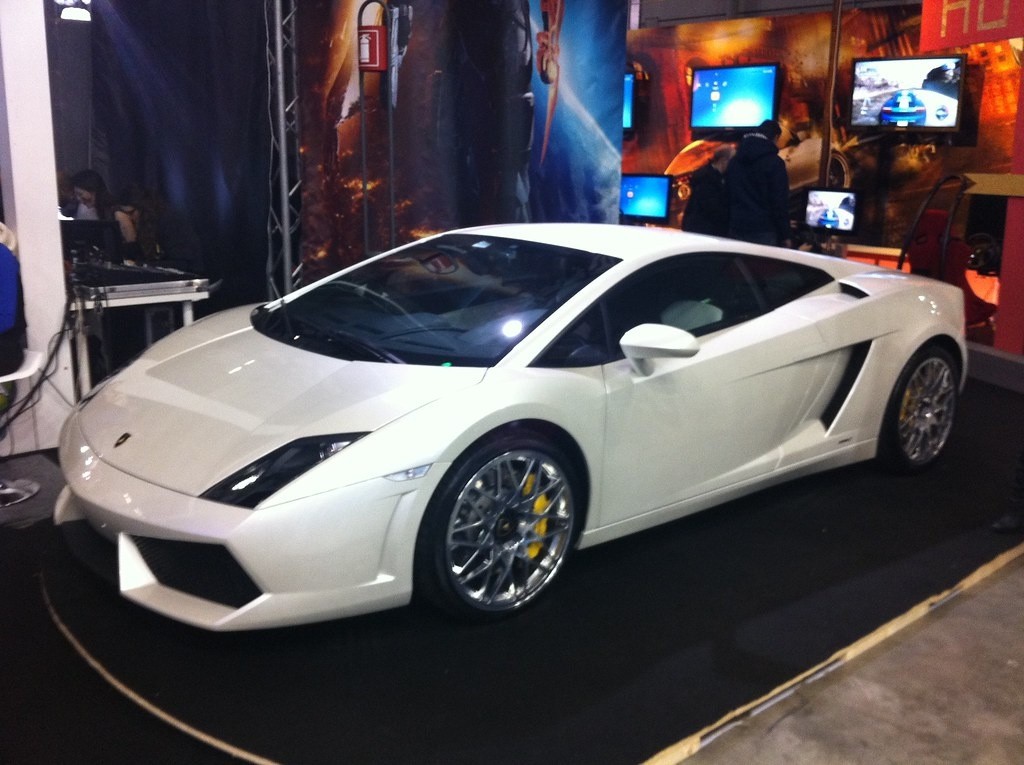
[74,169,132,264]
[681,147,738,238]
[722,119,793,250]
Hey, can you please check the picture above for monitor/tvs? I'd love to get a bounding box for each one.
[848,53,968,132]
[621,172,672,224]
[61,219,123,261]
[690,62,781,133]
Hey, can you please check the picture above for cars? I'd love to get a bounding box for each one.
[55,222,968,633]
[819,209,839,227]
[880,91,927,126]
